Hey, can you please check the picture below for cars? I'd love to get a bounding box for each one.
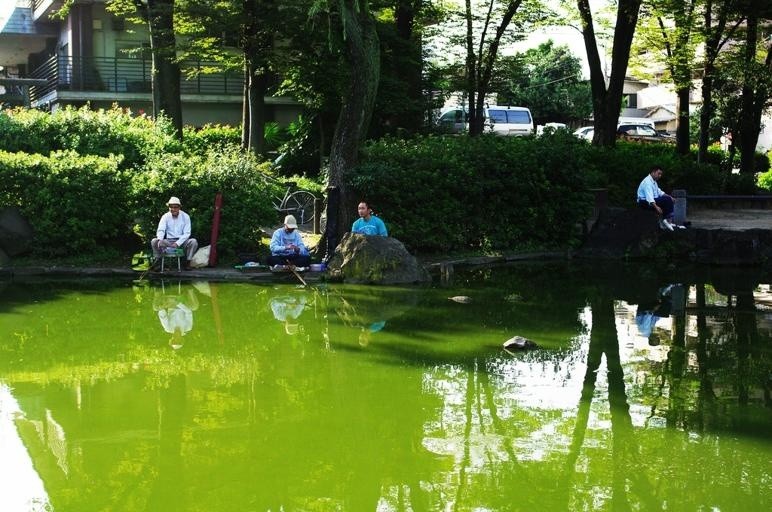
[536,123,566,139]
[573,126,595,140]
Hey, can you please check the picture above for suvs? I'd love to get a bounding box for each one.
[616,123,676,143]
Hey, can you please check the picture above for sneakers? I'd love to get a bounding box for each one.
[660,220,677,230]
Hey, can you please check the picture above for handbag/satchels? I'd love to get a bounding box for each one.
[191,244,212,268]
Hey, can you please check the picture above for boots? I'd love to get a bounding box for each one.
[184,260,193,271]
[153,259,162,272]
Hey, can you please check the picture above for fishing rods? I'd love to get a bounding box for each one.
[286,259,307,287]
[138,246,166,281]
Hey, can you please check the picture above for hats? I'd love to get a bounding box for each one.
[165,196,182,208]
[284,214,298,229]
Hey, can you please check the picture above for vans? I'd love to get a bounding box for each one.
[435,105,534,139]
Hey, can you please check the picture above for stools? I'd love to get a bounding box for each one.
[161,254,181,274]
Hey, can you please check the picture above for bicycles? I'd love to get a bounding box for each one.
[258,172,318,225]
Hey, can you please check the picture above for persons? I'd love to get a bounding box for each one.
[154,301,402,346]
[350,200,388,237]
[635,165,679,231]
[266,215,311,268]
[635,307,668,351]
[148,195,199,273]
